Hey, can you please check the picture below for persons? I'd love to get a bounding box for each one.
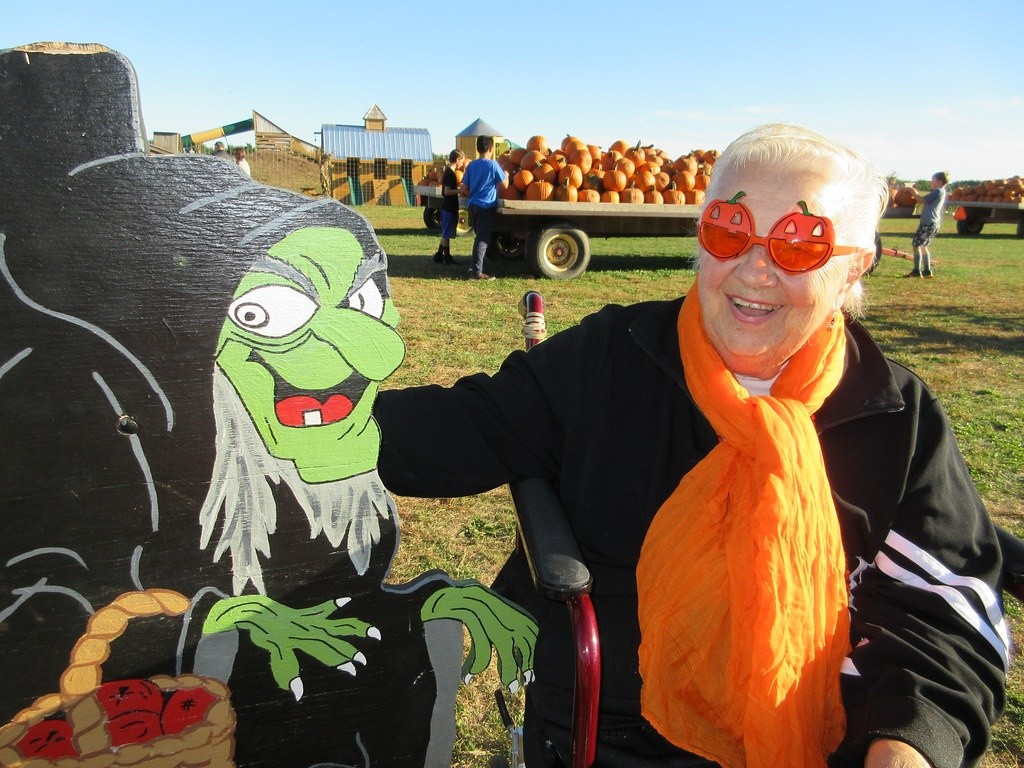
[460,135,510,280]
[372,121,1009,768]
[904,171,949,279]
[432,148,464,258]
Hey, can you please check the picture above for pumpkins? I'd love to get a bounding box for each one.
[418,160,472,187]
[946,178,1024,204]
[887,176,919,207]
[496,134,720,204]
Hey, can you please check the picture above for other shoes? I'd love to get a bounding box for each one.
[433,251,459,265]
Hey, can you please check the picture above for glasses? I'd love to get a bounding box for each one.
[694,190,864,276]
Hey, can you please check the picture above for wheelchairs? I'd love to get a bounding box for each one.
[494,290,1023,768]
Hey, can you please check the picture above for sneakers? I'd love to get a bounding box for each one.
[903,269,933,278]
[468,265,497,281]
